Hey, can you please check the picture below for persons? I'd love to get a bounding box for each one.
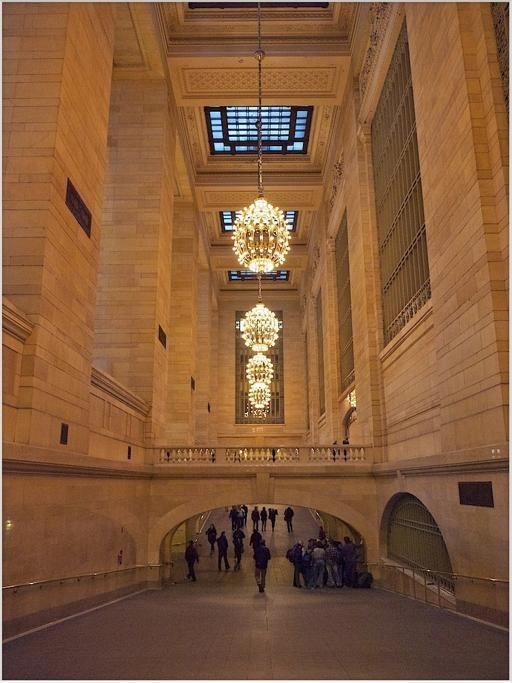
[333,440,337,445]
[343,437,349,444]
[184,505,365,593]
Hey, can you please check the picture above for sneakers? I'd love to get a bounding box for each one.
[253,527,293,533]
[258,585,265,593]
[293,581,345,591]
[183,575,196,581]
[217,565,240,572]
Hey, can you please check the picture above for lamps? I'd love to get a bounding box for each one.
[229,3,291,412]
